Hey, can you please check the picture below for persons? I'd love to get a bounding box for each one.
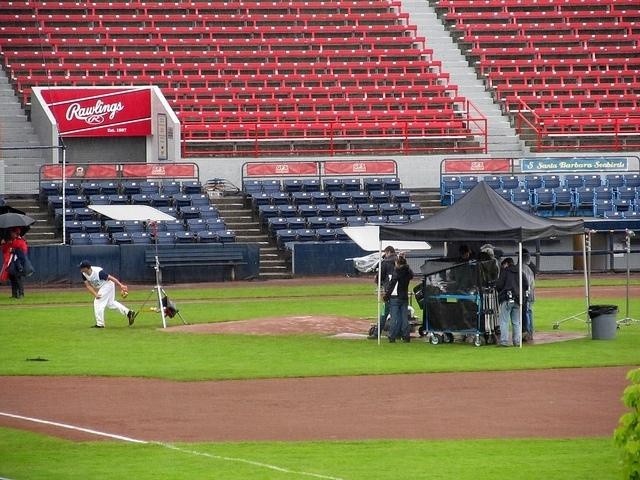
[77,260,136,329]
[0,206,32,239]
[375,247,537,343]
[1,227,35,300]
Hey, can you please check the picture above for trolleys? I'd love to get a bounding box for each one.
[368,287,501,347]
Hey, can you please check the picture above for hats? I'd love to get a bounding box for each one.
[77,260,91,267]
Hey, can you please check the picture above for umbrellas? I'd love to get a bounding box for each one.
[0,212,36,229]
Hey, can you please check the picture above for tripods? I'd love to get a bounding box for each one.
[130,256,189,329]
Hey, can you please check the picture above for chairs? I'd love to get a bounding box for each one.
[0,0,640,245]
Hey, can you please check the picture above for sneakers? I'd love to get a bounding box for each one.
[388,337,411,343]
[367,335,378,339]
[127,311,135,325]
[91,325,104,328]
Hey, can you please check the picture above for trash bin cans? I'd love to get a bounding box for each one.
[589,305,619,340]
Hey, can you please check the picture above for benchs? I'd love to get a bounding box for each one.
[144,252,246,283]
